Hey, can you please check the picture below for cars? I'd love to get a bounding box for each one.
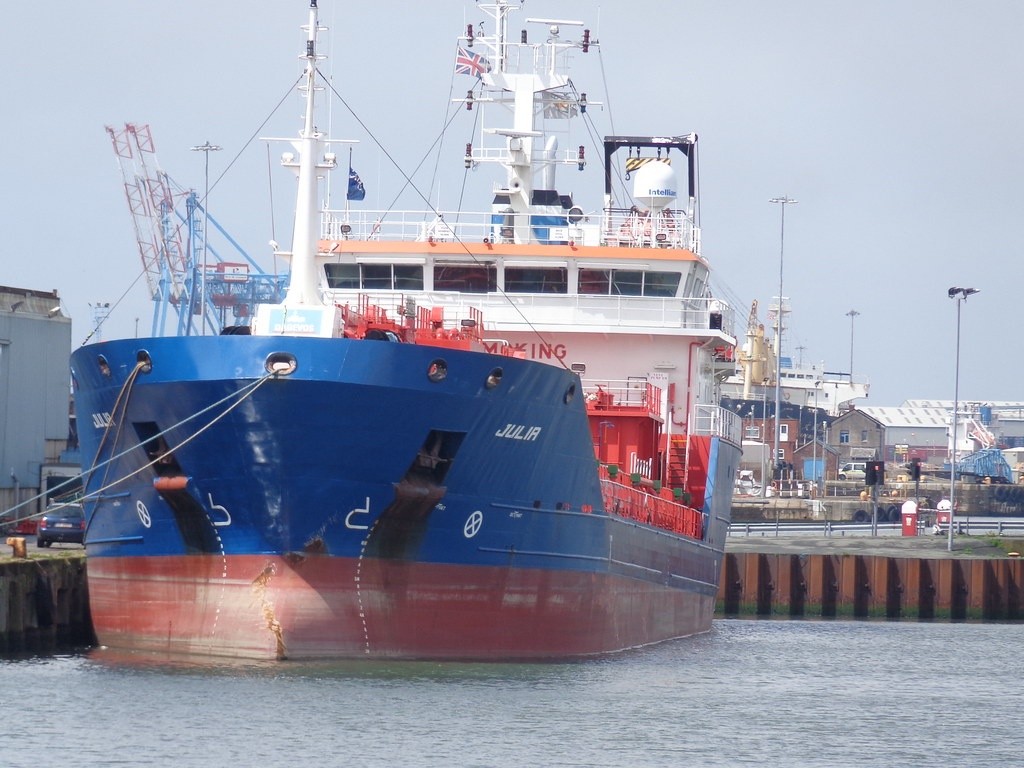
[36,500,87,548]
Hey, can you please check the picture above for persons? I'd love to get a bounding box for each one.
[775,462,794,480]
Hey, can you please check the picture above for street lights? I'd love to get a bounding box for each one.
[946,285,982,553]
[768,195,798,468]
[846,308,862,385]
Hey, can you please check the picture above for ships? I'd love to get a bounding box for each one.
[67,0,746,661]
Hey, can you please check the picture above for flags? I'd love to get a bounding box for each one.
[347,168,366,201]
[455,45,491,78]
[541,90,577,118]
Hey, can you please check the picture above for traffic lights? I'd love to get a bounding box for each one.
[904,462,913,479]
[866,461,876,484]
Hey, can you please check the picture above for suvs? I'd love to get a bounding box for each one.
[838,463,867,480]
